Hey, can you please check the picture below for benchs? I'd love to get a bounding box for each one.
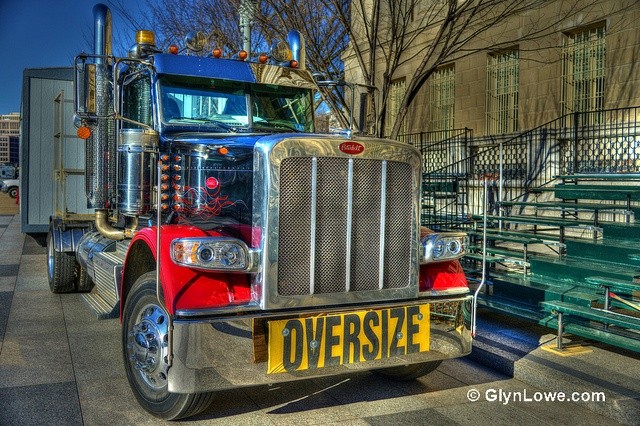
[461,254,505,293]
[552,173,640,185]
[529,185,640,211]
[467,229,544,278]
[537,301,640,354]
[584,275,639,311]
[471,212,596,242]
[498,200,640,222]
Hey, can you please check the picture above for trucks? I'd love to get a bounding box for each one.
[19,4,488,422]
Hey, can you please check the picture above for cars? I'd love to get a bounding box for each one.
[1,175,19,198]
